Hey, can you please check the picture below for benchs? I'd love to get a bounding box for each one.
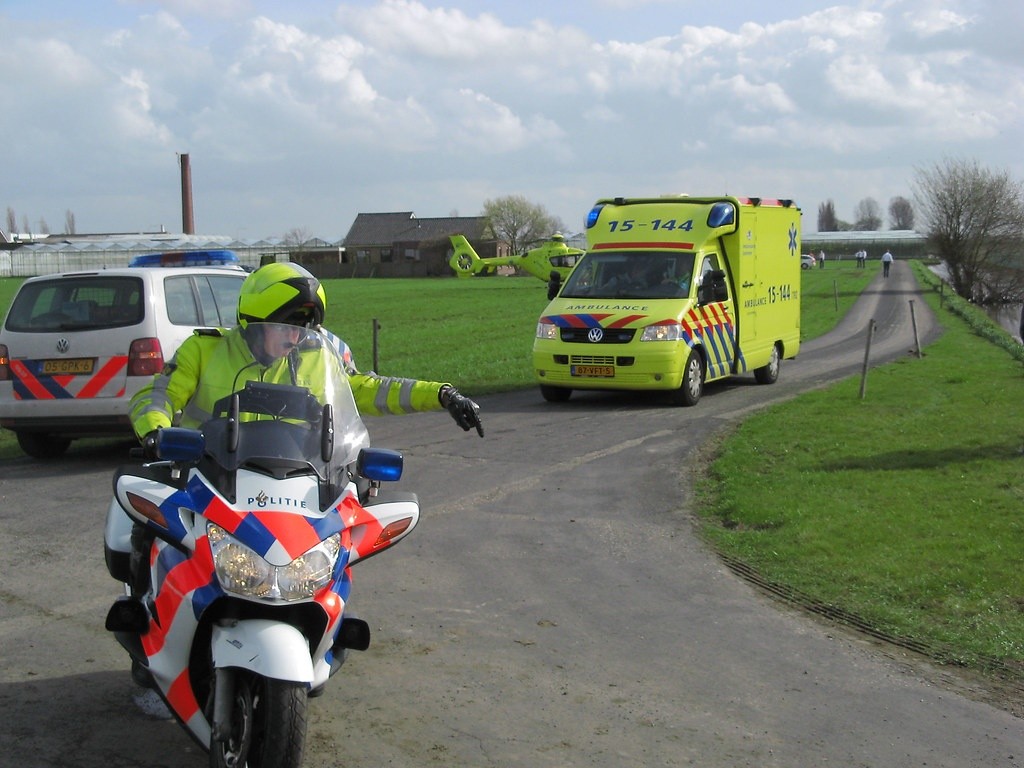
[32,300,140,325]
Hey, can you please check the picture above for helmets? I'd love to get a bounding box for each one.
[236,261,327,338]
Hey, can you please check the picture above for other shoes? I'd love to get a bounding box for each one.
[131,657,154,689]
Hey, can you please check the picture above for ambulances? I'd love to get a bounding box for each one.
[531,194,803,407]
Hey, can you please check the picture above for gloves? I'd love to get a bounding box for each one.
[442,384,486,439]
[143,432,164,469]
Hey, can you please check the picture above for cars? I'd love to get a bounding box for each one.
[800,254,817,271]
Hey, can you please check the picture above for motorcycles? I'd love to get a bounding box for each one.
[103,323,425,768]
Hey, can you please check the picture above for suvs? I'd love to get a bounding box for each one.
[0,249,361,463]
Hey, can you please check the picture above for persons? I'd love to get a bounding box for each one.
[818,250,826,268]
[855,249,867,269]
[881,249,894,277]
[127,261,486,474]
[662,255,692,294]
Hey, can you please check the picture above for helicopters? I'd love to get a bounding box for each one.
[448,226,588,290]
